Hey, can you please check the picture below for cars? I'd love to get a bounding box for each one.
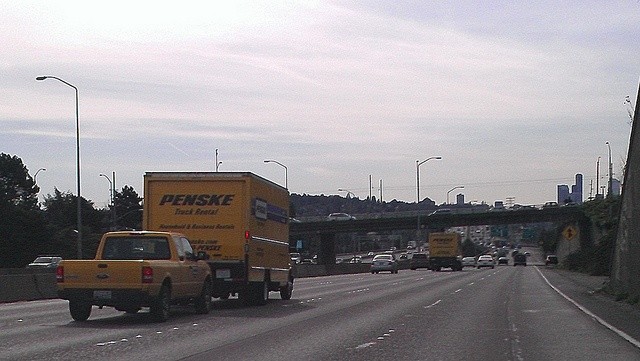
[301,258,315,264]
[542,201,558,209]
[351,258,361,263]
[461,256,475,267]
[498,257,508,265]
[477,254,494,269]
[327,212,357,220]
[371,254,399,273]
[400,252,408,257]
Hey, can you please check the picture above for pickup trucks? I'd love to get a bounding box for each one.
[56,228,212,320]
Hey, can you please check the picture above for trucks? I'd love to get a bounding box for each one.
[141,169,294,305]
[429,231,463,270]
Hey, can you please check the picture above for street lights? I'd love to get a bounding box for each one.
[605,141,612,196]
[99,172,113,208]
[36,74,80,259]
[597,156,601,194]
[264,159,288,189]
[416,155,442,202]
[447,184,465,204]
[33,167,47,191]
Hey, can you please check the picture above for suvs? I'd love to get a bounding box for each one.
[514,253,526,266]
[29,255,61,266]
[546,255,558,265]
[410,254,429,269]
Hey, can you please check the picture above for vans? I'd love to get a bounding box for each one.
[432,208,451,215]
[290,251,300,261]
